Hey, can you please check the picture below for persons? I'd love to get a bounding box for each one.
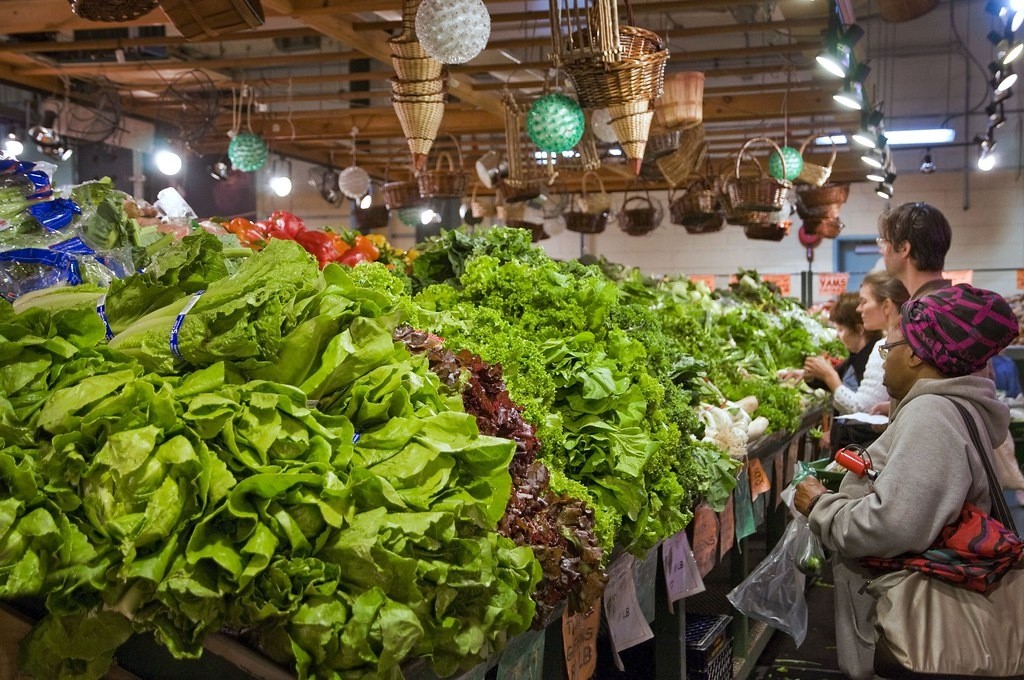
[776,289,886,423]
[876,201,998,383]
[794,284,1024,680]
[802,269,910,415]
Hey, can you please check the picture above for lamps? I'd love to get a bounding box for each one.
[816,0,1024,200]
[0,110,485,226]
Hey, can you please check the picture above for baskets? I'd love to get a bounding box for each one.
[350,1,854,239]
[70,0,159,22]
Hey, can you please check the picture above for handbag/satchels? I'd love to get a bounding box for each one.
[857,392,1024,680]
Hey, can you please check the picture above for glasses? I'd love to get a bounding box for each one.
[875,237,892,248]
[879,339,907,360]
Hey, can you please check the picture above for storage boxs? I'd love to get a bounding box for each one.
[629,612,737,680]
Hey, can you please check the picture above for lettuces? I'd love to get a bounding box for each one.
[331,225,848,619]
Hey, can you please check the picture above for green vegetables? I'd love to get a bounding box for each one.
[0,171,229,302]
[0,241,540,680]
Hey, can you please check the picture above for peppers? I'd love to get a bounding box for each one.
[209,211,421,276]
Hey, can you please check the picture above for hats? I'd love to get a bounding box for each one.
[900,282,1020,377]
